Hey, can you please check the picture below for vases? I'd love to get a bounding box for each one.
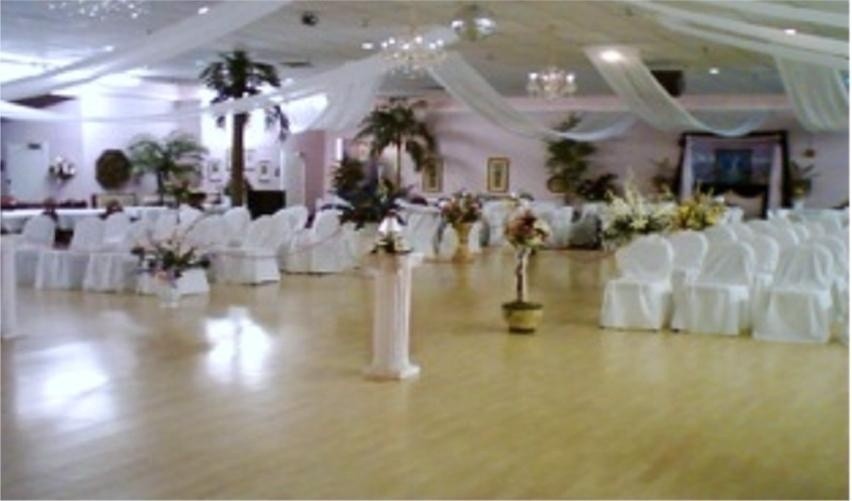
[502,304,543,333]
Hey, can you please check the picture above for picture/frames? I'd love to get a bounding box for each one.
[487,157,509,193]
[422,157,443,193]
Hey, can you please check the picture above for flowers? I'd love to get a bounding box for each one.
[603,166,730,249]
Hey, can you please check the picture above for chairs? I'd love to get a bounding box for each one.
[600,208,849,343]
